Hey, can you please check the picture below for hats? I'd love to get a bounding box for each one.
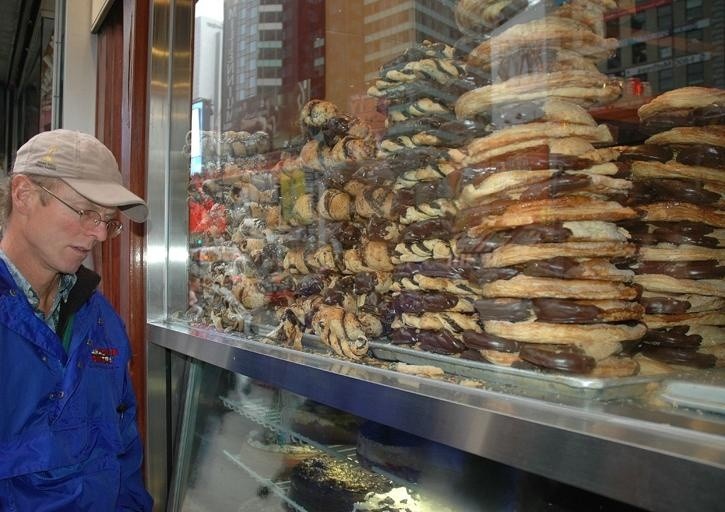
[12,129,149,223]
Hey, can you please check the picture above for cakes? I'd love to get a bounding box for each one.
[240,380,454,512]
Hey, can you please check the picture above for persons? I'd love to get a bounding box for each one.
[0,129,154,511]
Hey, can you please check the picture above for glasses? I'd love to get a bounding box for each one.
[40,183,123,239]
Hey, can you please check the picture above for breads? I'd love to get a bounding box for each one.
[187,0,725,379]
